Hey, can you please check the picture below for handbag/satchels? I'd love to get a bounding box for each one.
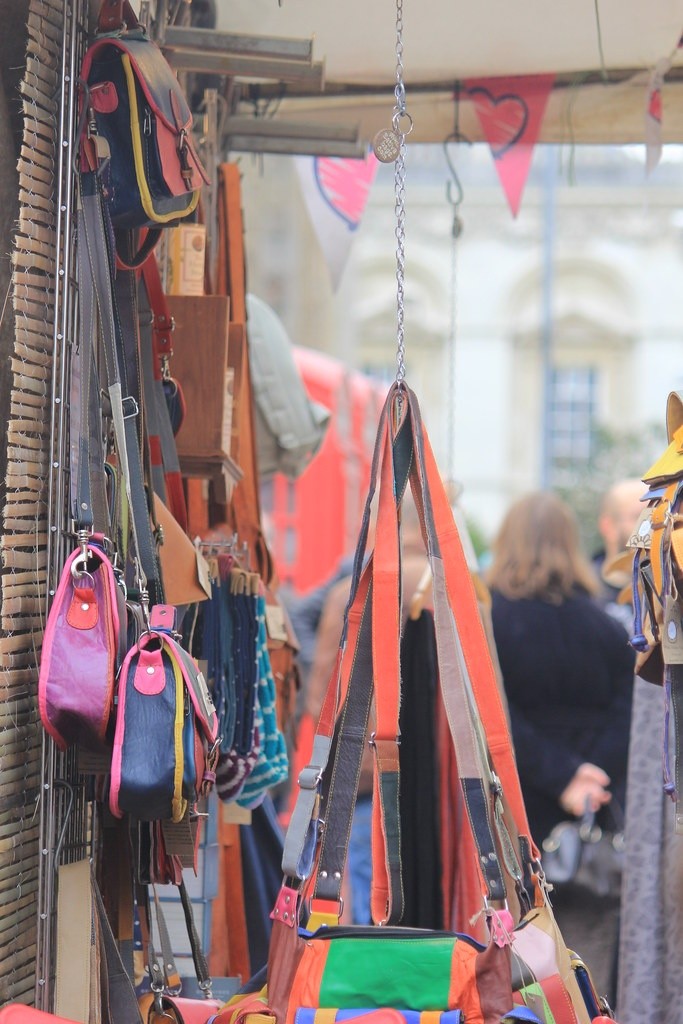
[144,884,617,1024]
[107,604,223,824]
[161,375,188,438]
[538,795,626,901]
[71,0,211,273]
[37,542,130,753]
[253,536,301,736]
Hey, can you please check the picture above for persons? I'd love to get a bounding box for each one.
[310,485,467,727]
[272,550,367,714]
[590,479,654,641]
[489,494,636,1006]
